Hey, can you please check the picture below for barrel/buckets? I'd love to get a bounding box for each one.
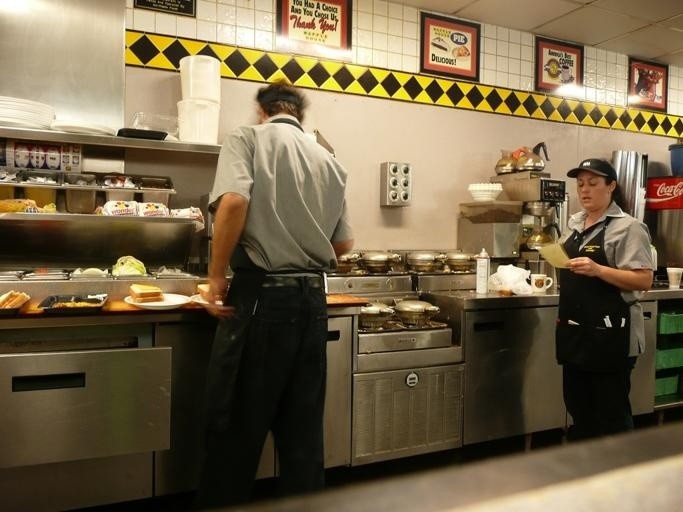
[176,99,220,147]
[179,54,222,104]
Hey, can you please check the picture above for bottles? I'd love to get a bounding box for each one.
[477,250,491,295]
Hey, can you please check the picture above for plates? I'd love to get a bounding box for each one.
[2,294,224,316]
[116,128,168,143]
[0,92,116,136]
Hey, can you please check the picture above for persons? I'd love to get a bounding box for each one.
[538,156,654,440]
[195,80,355,512]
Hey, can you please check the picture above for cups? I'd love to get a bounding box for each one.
[177,55,223,145]
[667,267,682,288]
[531,273,552,293]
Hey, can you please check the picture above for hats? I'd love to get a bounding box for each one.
[567,159,617,181]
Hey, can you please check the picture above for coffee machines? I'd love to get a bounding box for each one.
[490,171,566,260]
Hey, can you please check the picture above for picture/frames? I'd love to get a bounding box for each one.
[420,11,481,82]
[628,56,668,112]
[535,36,584,91]
[134,0,196,18]
[276,0,352,58]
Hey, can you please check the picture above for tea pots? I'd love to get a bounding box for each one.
[494,141,552,173]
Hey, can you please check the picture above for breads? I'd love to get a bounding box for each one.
[129,283,164,302]
[197,283,222,300]
[0,290,31,309]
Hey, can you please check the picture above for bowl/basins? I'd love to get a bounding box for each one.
[468,183,501,202]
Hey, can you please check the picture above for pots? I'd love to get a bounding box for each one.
[334,251,477,331]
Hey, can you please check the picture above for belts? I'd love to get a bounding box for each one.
[261,273,324,289]
